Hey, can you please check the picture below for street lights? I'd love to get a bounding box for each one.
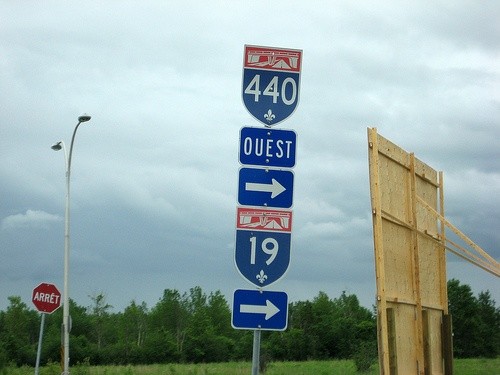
[51,115,91,374]
